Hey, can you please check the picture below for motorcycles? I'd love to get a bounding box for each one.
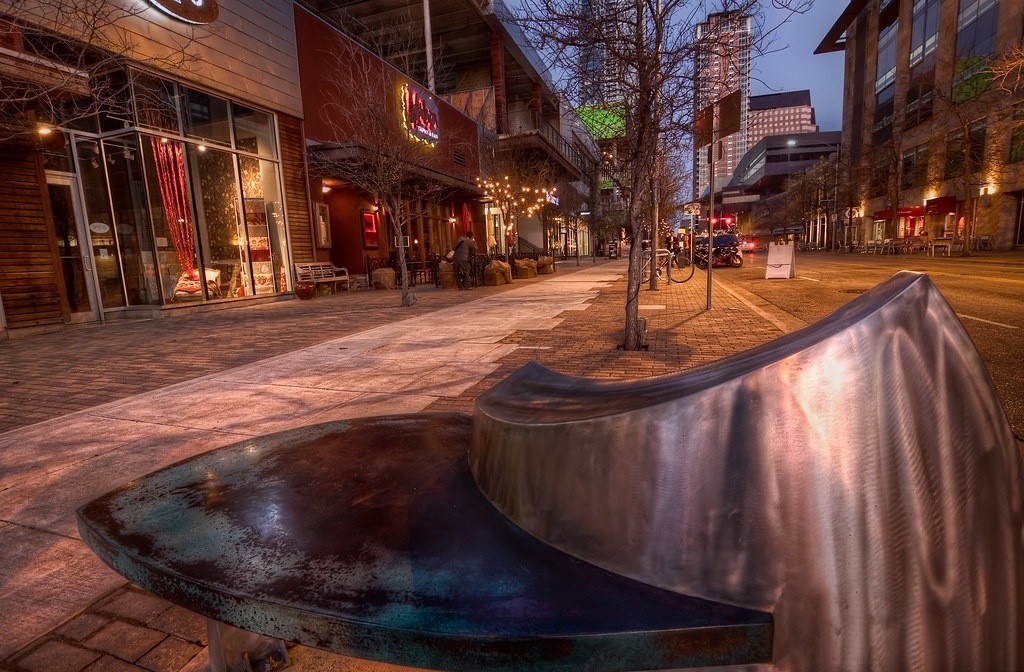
[694,240,744,269]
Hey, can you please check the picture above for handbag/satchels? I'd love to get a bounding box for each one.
[443,249,455,263]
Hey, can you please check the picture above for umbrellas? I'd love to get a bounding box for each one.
[462,201,471,240]
[487,207,498,254]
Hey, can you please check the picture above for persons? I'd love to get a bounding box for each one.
[852,239,858,251]
[451,231,478,291]
[641,241,647,251]
[919,227,925,236]
[665,226,696,268]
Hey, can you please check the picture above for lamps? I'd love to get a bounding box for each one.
[322,185,332,193]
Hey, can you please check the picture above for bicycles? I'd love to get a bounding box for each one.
[642,248,695,284]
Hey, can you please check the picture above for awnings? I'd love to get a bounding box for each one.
[499,127,582,183]
[873,195,956,217]
[306,139,490,204]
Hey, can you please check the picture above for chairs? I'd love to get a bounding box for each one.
[795,242,826,252]
[430,251,542,289]
[411,265,427,283]
[837,238,992,257]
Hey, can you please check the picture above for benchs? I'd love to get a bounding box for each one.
[293,262,350,297]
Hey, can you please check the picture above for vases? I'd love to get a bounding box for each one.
[295,281,314,301]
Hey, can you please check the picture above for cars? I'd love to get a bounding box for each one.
[712,234,743,258]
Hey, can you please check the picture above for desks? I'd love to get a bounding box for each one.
[927,238,952,257]
